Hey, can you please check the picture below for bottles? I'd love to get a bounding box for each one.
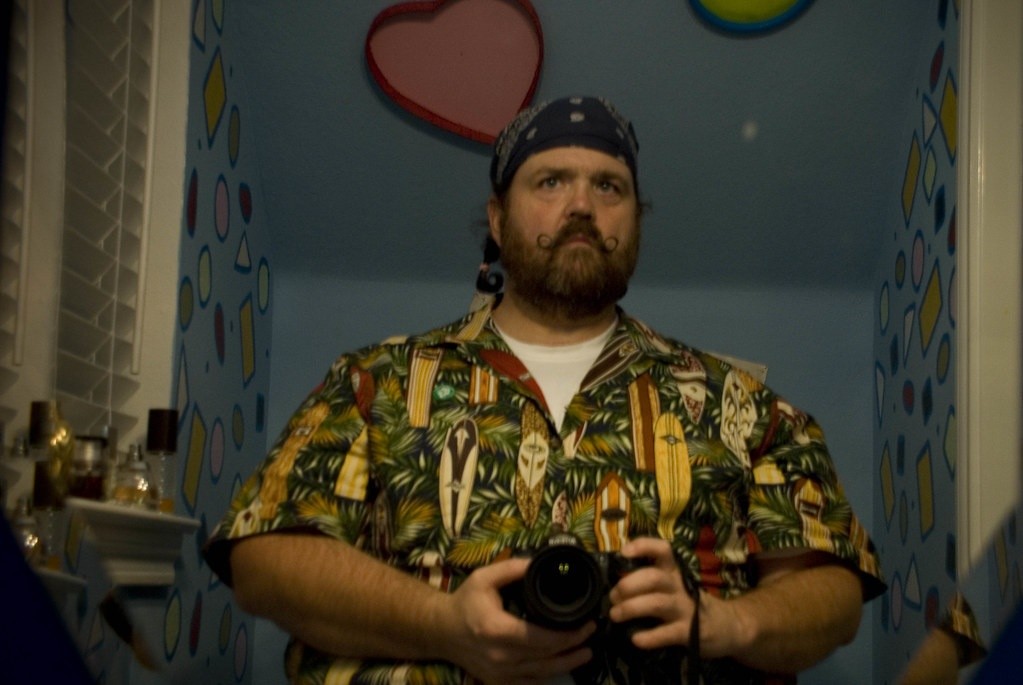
[7,408,179,571]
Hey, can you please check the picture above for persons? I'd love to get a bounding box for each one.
[202,98,889,685]
[898,594,986,685]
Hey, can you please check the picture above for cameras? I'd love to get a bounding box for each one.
[498,531,665,654]
